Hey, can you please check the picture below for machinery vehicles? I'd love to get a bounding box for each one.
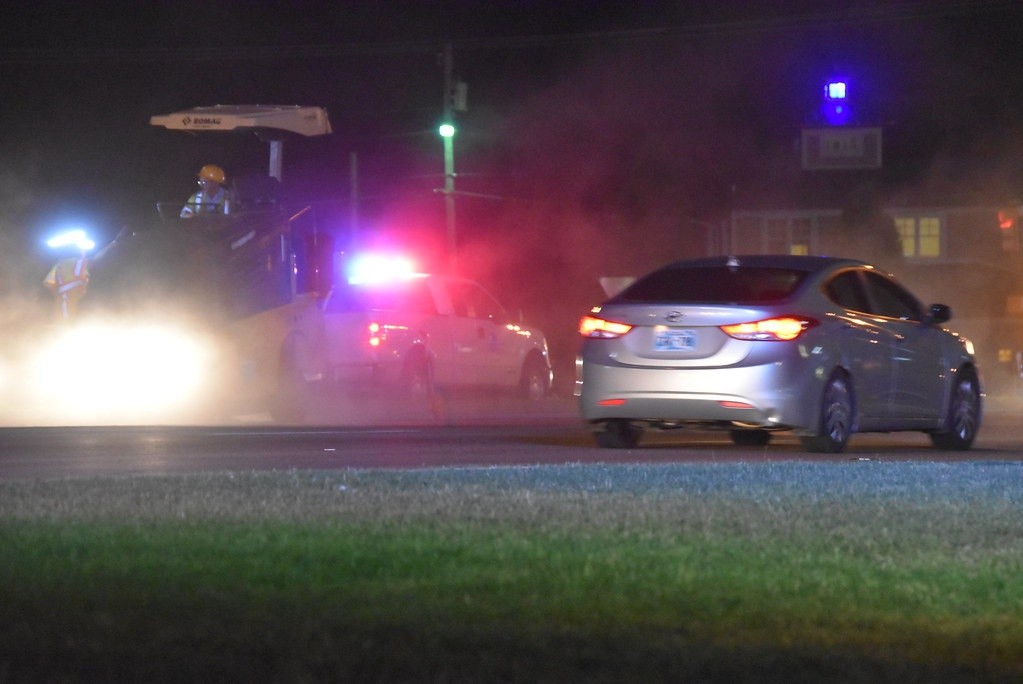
[47,99,333,414]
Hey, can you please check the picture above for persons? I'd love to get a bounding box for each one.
[42,245,90,329]
[180,164,238,219]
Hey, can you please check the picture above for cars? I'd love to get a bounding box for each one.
[282,272,554,407]
[577,253,984,457]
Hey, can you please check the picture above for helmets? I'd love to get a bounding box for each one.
[197,164,224,184]
